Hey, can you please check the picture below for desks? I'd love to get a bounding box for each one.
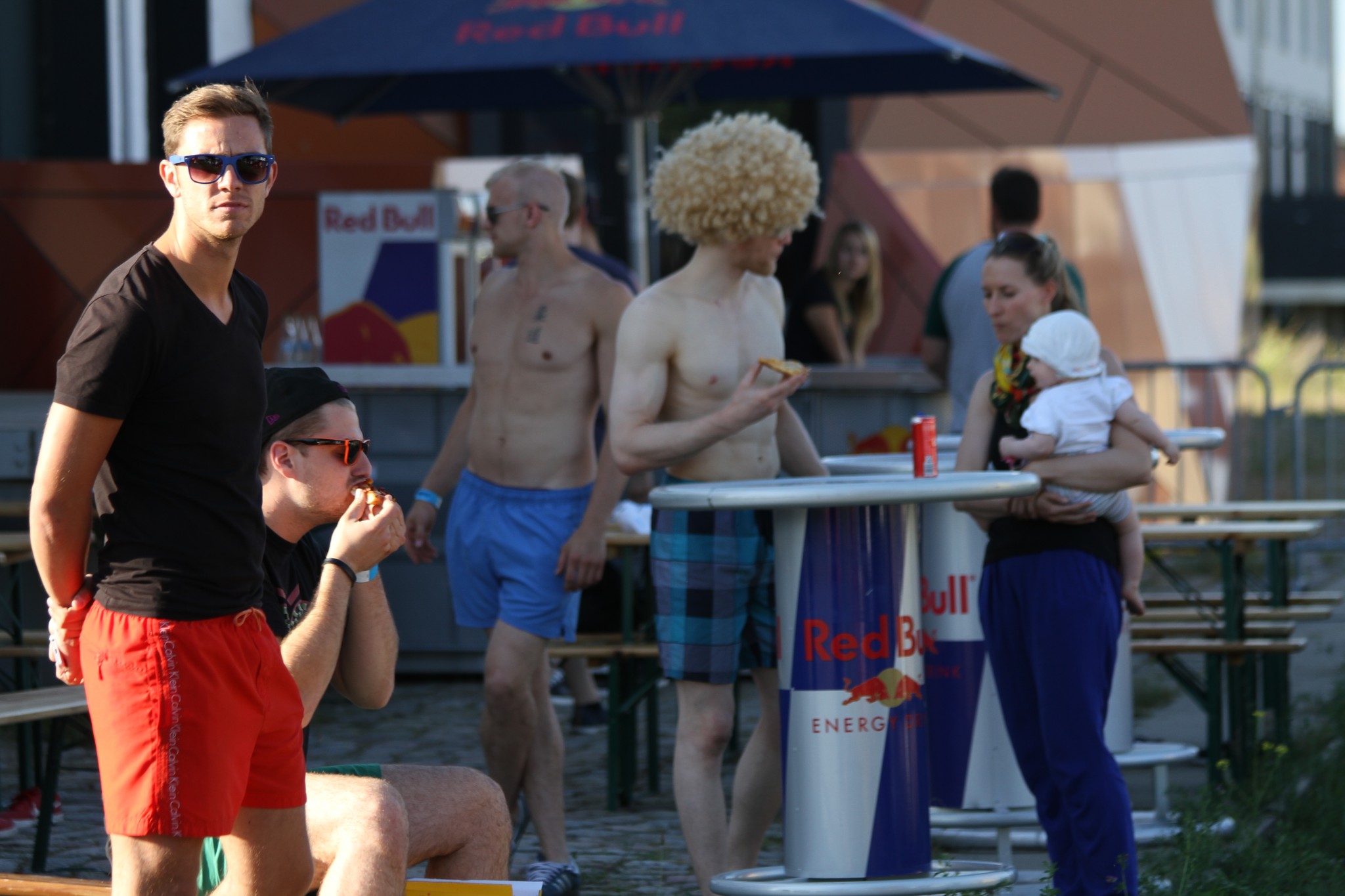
[602,425,1345,896]
[0,500,51,800]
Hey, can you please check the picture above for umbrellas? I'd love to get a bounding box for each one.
[168,0,1060,299]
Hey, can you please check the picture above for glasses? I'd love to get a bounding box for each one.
[169,153,275,184]
[486,202,549,224]
[283,437,371,466]
[996,229,1057,253]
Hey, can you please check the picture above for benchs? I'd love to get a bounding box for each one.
[0,686,87,876]
[543,593,1344,713]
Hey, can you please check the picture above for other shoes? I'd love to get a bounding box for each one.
[550,666,610,707]
[559,702,609,734]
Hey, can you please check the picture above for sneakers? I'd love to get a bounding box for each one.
[526,853,581,896]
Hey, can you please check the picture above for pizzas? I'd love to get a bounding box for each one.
[757,357,811,377]
[351,478,396,505]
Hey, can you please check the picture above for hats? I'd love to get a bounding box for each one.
[263,367,352,447]
[1021,309,1106,378]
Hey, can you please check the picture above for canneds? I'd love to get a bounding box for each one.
[911,414,937,478]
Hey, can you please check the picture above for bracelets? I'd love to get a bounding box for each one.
[322,557,357,588]
[412,486,443,511]
[354,563,379,583]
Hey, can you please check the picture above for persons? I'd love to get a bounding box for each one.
[924,168,1089,436]
[404,159,640,895]
[260,366,513,896]
[28,84,316,895]
[610,112,829,896]
[785,221,884,368]
[951,230,1181,896]
